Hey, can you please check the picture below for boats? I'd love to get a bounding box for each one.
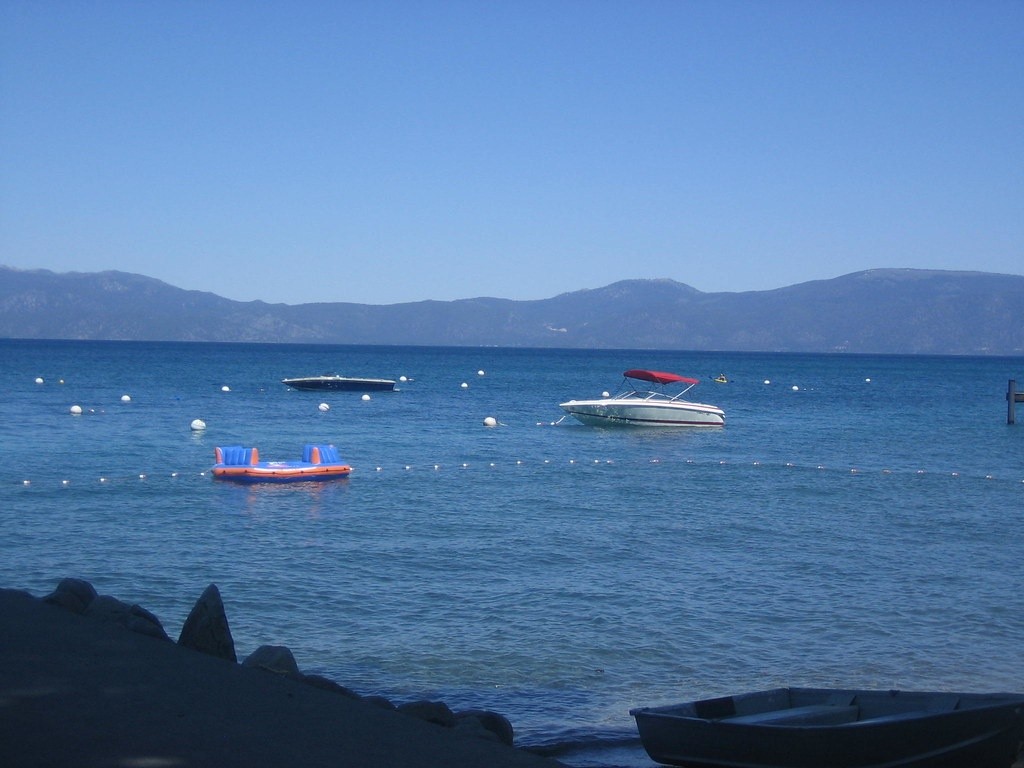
[281,377,396,392]
[559,369,725,428]
[715,378,727,383]
[628,686,1024,768]
[211,444,351,484]
